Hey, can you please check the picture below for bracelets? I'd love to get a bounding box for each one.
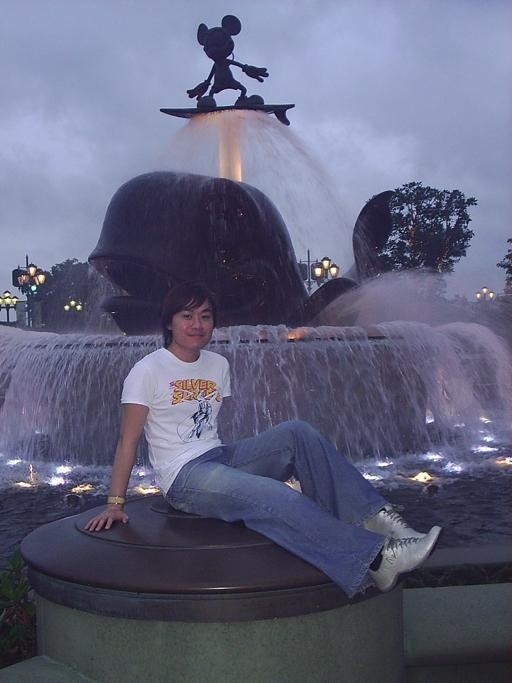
[103,495,130,507]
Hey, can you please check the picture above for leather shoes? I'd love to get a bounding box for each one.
[371,526,443,592]
[362,506,426,539]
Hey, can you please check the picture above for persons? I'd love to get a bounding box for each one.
[83,279,448,600]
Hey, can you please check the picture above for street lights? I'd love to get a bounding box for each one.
[62,298,84,316]
[313,255,339,283]
[475,285,494,303]
[1,289,19,323]
[16,254,47,326]
[296,247,319,296]
